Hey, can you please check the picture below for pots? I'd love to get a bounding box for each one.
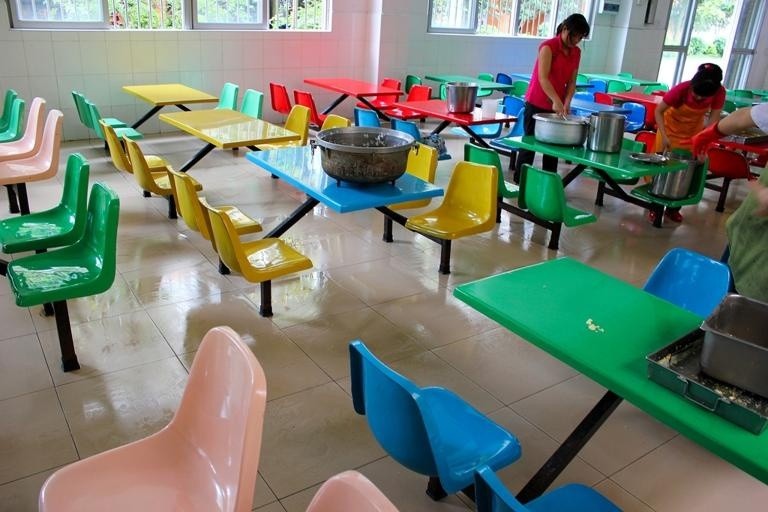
[444,80,479,114]
[310,125,420,190]
[647,150,703,201]
[583,110,628,155]
[531,111,589,146]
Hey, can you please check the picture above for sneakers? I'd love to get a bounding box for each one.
[647,209,683,225]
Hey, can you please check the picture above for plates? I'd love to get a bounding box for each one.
[629,151,666,165]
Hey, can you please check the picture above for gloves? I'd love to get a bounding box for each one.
[679,119,729,158]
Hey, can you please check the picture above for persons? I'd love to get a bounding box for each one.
[678,101,768,302]
[514,12,588,186]
[648,62,726,225]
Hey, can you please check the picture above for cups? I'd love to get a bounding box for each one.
[480,98,499,120]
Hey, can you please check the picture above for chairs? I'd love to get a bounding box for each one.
[0,90,65,216]
[167,164,312,316]
[1,152,119,372]
[271,73,768,160]
[643,248,732,320]
[219,83,263,118]
[284,104,352,142]
[306,469,398,512]
[704,149,748,211]
[38,326,267,512]
[463,137,708,251]
[384,142,498,274]
[98,120,202,218]
[349,340,620,512]
[71,91,143,155]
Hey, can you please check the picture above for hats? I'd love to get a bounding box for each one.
[691,62,723,99]
